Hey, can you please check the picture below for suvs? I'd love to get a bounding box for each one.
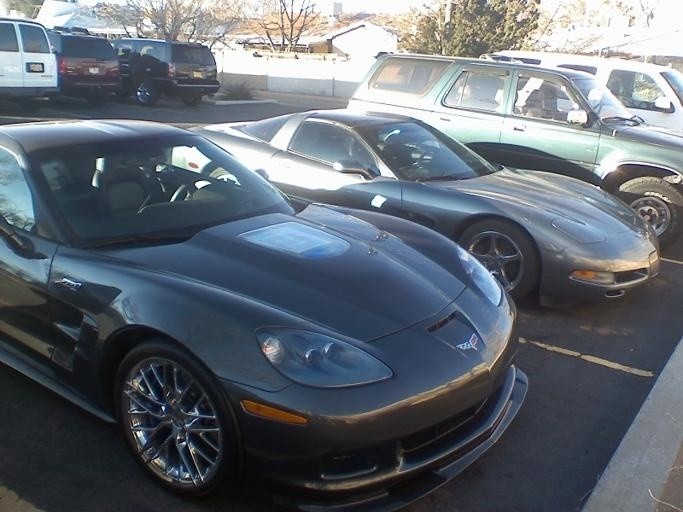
[344,50,682,248]
[106,36,219,108]
[0,17,61,111]
[44,24,124,107]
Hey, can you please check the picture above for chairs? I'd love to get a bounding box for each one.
[99,144,157,223]
[522,90,545,117]
[612,81,628,104]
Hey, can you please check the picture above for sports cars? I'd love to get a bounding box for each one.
[0,115,529,511]
[167,103,665,310]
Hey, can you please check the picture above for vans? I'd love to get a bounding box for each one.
[457,50,683,143]
